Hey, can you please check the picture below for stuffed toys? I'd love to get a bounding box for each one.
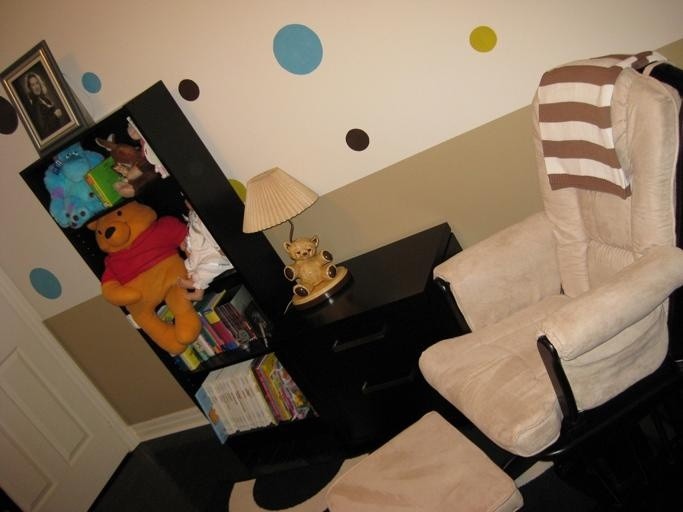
[125,114,170,182]
[93,132,155,195]
[41,142,109,227]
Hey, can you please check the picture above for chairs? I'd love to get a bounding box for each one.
[408,58,680,469]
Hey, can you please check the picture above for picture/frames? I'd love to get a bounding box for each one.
[0,39,89,160]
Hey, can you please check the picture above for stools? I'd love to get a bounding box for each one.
[325,411,524,512]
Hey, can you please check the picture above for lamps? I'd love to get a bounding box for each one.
[241,165,353,313]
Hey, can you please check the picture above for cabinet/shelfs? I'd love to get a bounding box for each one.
[276,222,465,450]
[15,80,357,476]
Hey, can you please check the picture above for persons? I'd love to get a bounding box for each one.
[176,186,239,302]
[86,198,201,353]
[21,71,63,136]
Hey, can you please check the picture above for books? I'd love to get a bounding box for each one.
[156,285,318,447]
[83,156,125,207]
